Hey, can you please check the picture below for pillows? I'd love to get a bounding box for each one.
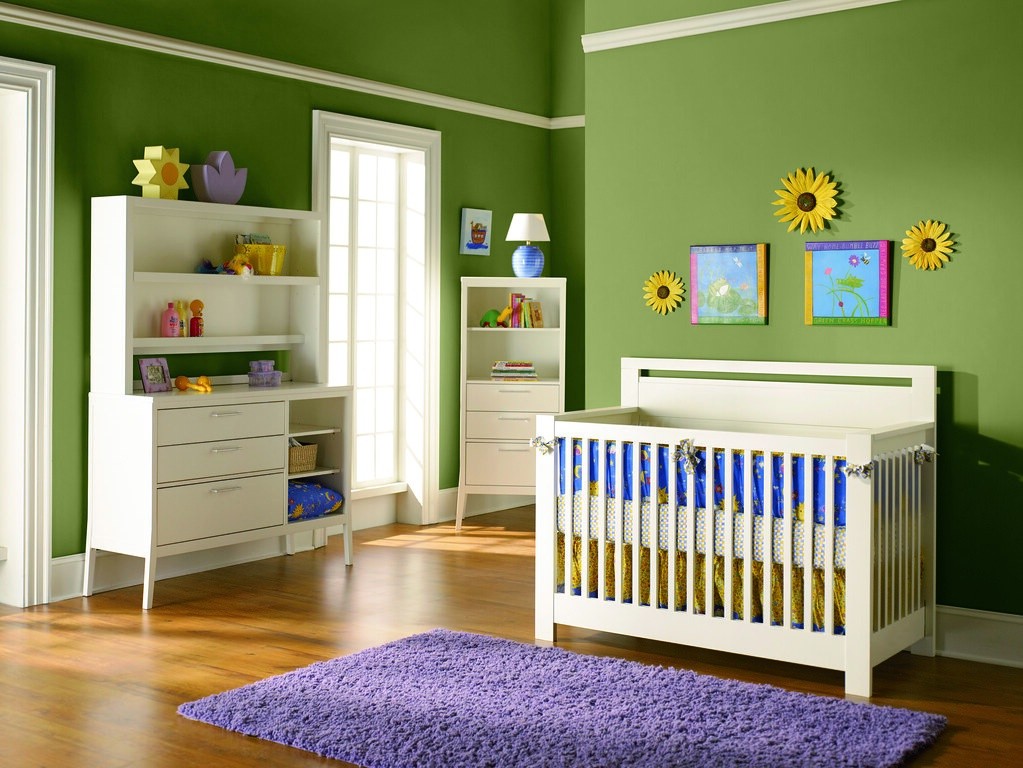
[288,481,343,522]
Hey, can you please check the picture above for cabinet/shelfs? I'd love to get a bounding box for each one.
[82,195,355,610]
[454,276,568,530]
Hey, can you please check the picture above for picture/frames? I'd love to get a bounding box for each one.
[138,357,173,393]
[690,244,769,325]
[804,241,892,327]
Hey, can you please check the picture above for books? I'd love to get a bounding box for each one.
[509,293,543,328]
[490,360,539,381]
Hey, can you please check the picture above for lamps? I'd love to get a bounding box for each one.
[504,213,551,278]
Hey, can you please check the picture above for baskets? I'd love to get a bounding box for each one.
[289,437,317,474]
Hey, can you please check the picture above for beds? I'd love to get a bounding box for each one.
[535,356,936,700]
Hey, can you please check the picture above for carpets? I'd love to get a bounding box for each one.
[176,627,950,768]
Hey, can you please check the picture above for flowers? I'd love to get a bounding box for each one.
[770,167,840,235]
[899,219,957,272]
[642,271,686,317]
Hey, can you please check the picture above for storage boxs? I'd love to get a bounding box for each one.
[249,360,282,388]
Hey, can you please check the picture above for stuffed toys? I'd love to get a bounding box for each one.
[480,306,512,328]
